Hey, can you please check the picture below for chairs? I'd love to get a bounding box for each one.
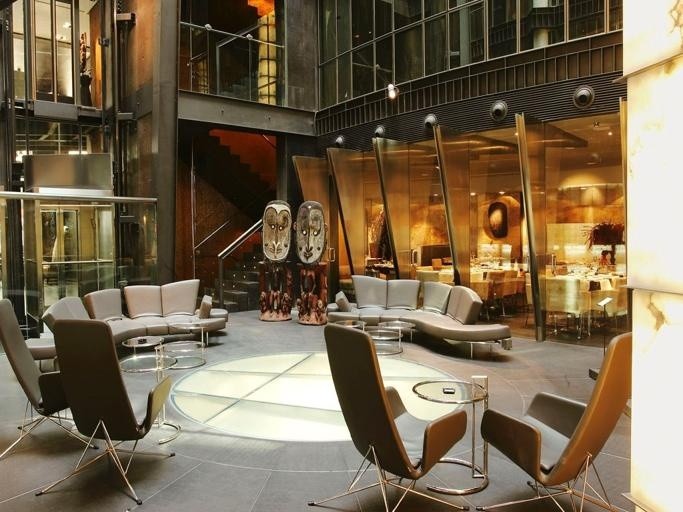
[22,280,228,374]
[363,243,628,340]
[480,331,634,512]
[33,318,172,507]
[1,293,99,462]
[320,323,470,512]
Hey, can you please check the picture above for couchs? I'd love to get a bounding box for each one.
[325,275,513,359]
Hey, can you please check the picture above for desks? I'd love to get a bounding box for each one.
[117,354,181,447]
